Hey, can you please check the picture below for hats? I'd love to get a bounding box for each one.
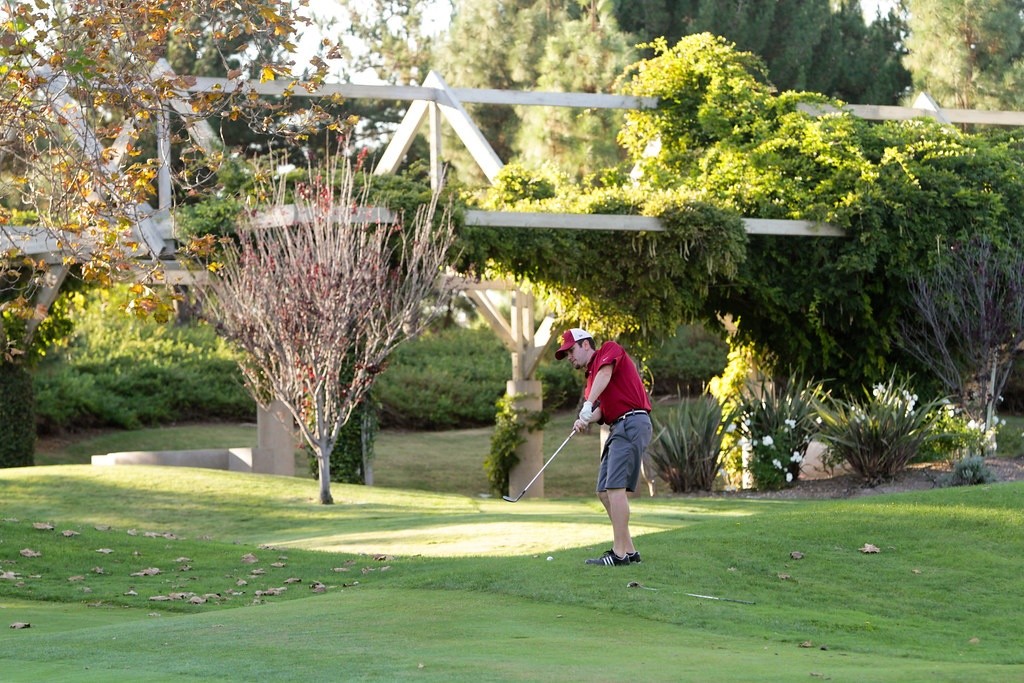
[555,328,593,360]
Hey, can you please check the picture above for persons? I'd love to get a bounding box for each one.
[555,327,652,568]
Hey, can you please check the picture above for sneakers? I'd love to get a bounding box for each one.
[585,552,630,566]
[599,549,641,563]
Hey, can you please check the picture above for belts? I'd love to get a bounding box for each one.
[609,409,648,426]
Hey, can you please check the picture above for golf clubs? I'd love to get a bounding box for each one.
[502,428,580,503]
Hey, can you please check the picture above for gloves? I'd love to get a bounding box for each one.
[579,401,594,422]
